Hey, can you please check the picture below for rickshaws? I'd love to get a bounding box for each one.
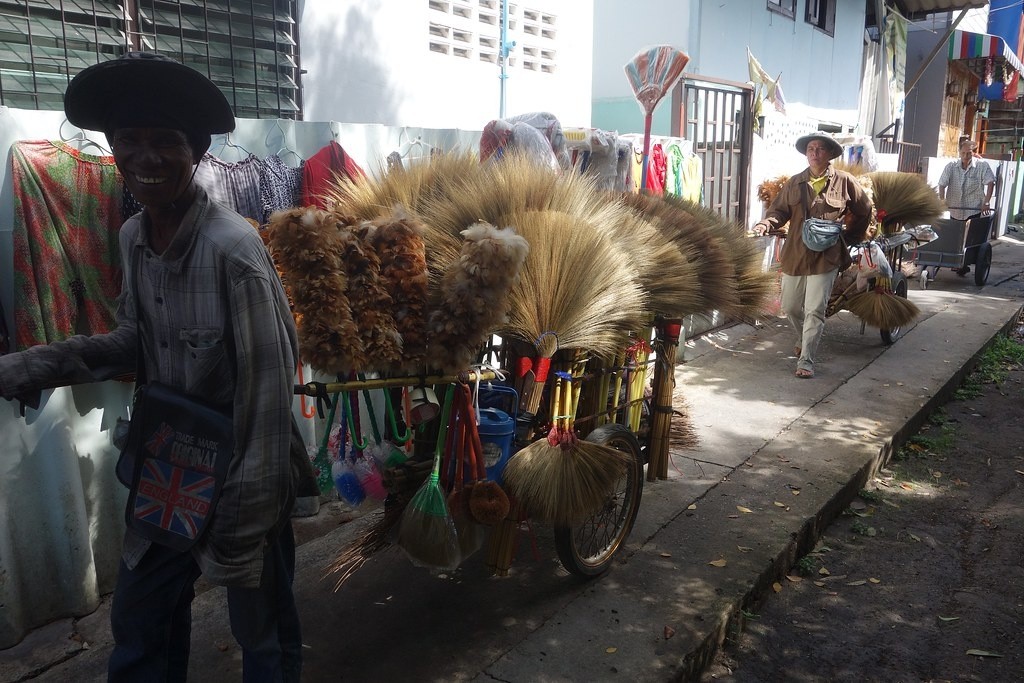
[292,337,655,578]
[755,226,909,345]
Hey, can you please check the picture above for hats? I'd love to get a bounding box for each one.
[796,130,843,161]
[64,50,236,134]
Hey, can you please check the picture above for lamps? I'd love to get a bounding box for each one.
[965,88,977,106]
[976,97,987,113]
[866,24,880,43]
[947,77,960,98]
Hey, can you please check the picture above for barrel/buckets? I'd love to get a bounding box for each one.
[447,384,522,486]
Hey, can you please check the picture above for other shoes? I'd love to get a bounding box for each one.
[952,266,970,274]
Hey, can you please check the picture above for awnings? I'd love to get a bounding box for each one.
[949,29,1024,86]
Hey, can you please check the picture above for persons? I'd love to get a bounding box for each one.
[1,52,322,683]
[938,141,996,276]
[750,130,872,377]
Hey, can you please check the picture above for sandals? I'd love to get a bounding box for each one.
[794,358,816,378]
[793,339,802,358]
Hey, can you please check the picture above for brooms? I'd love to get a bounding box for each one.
[834,158,949,334]
[313,43,789,572]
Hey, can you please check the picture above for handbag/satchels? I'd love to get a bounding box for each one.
[800,183,842,253]
[116,381,236,554]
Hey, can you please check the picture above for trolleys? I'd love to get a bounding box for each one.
[915,207,998,289]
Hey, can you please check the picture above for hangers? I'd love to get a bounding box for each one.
[398,126,433,158]
[208,131,251,156]
[60,117,112,157]
[274,118,303,160]
[329,119,340,141]
